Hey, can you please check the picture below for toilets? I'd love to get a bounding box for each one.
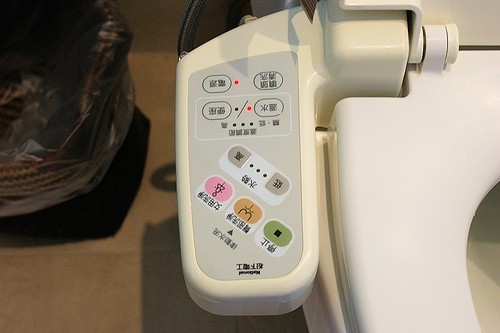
[178,0,500,333]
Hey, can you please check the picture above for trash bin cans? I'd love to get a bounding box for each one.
[0,0,152,242]
